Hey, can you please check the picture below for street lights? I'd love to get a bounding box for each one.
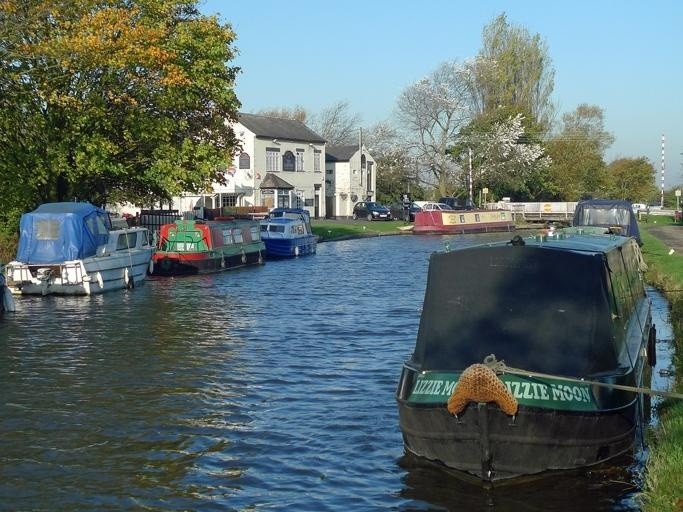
[361,134,376,199]
[458,147,473,207]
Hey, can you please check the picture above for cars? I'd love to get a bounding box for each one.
[353,197,476,224]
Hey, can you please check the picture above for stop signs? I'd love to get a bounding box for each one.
[543,203,550,212]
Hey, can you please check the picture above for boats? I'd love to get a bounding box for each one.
[569,196,643,253]
[392,226,657,489]
[410,208,515,236]
